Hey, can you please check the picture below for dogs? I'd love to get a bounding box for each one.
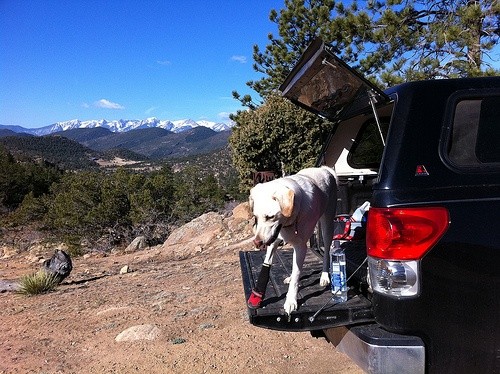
[247,165,339,313]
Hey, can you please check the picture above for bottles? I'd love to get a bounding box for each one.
[329,241,347,302]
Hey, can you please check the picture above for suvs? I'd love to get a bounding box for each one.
[239,37,500,374]
